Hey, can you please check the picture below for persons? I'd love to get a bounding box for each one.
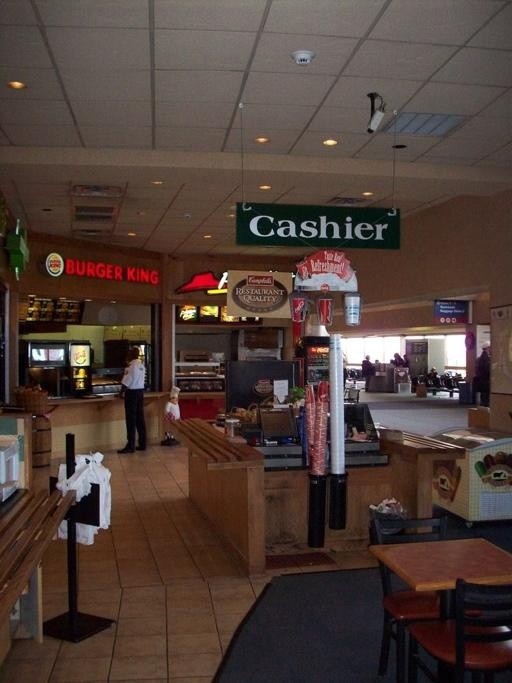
[391,352,404,368]
[361,354,374,392]
[476,341,490,406]
[160,385,183,439]
[401,354,409,367]
[113,347,149,453]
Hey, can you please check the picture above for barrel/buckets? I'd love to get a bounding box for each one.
[31,414,52,467]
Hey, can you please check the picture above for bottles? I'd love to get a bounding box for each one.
[215,414,226,426]
[224,418,241,441]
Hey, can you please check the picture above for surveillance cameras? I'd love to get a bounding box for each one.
[367,109,385,133]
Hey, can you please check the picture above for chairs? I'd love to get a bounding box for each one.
[409,579,512,682]
[369,513,483,683]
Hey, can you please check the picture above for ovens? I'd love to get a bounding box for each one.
[178,350,214,373]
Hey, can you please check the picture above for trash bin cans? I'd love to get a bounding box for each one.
[369,505,408,544]
[458,383,466,401]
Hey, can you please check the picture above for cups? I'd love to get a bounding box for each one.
[291,297,307,322]
[316,298,333,326]
[304,333,346,476]
[344,294,363,328]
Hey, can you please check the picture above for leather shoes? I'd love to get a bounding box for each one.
[134,446,146,451]
[116,447,136,454]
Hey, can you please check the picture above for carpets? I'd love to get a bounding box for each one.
[211,566,511,682]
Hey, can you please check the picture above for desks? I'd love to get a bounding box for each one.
[369,537,512,682]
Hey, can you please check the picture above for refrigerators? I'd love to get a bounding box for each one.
[488,303,512,436]
[424,425,512,522]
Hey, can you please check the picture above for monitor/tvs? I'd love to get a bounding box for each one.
[345,404,367,435]
[259,407,297,438]
